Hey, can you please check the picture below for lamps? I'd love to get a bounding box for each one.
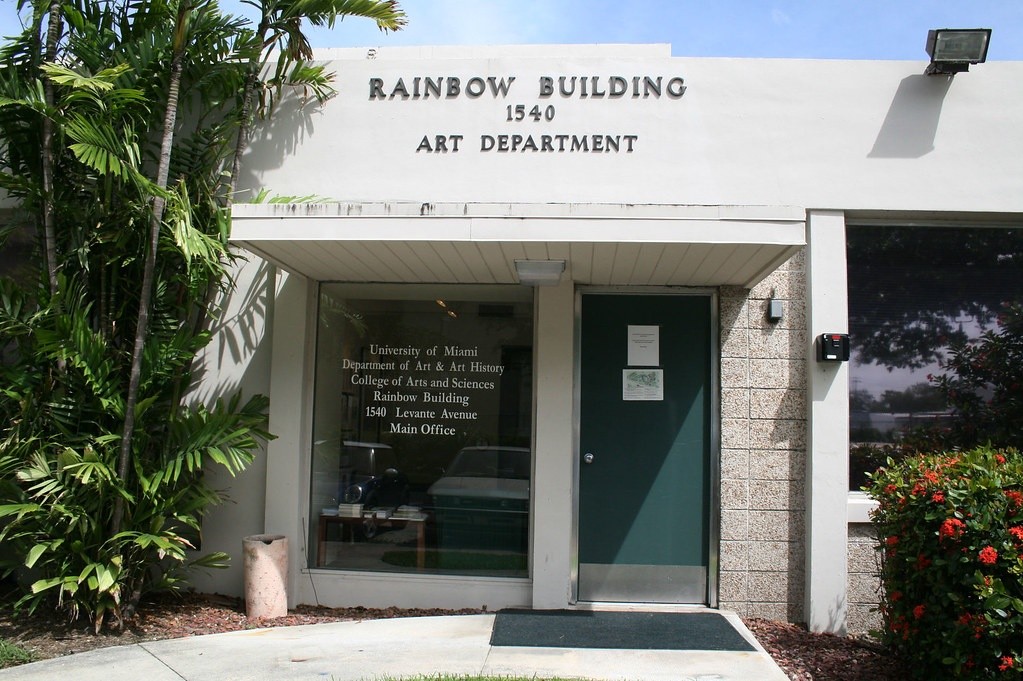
[926,27,992,75]
[514,258,567,288]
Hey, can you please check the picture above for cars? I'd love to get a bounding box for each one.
[308,439,409,542]
[424,445,532,549]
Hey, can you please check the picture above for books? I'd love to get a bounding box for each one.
[319,504,428,521]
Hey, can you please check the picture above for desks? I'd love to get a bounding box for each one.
[318,510,433,574]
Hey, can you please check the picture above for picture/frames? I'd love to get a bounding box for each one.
[356,346,384,443]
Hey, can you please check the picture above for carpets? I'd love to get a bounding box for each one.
[489,608,760,652]
